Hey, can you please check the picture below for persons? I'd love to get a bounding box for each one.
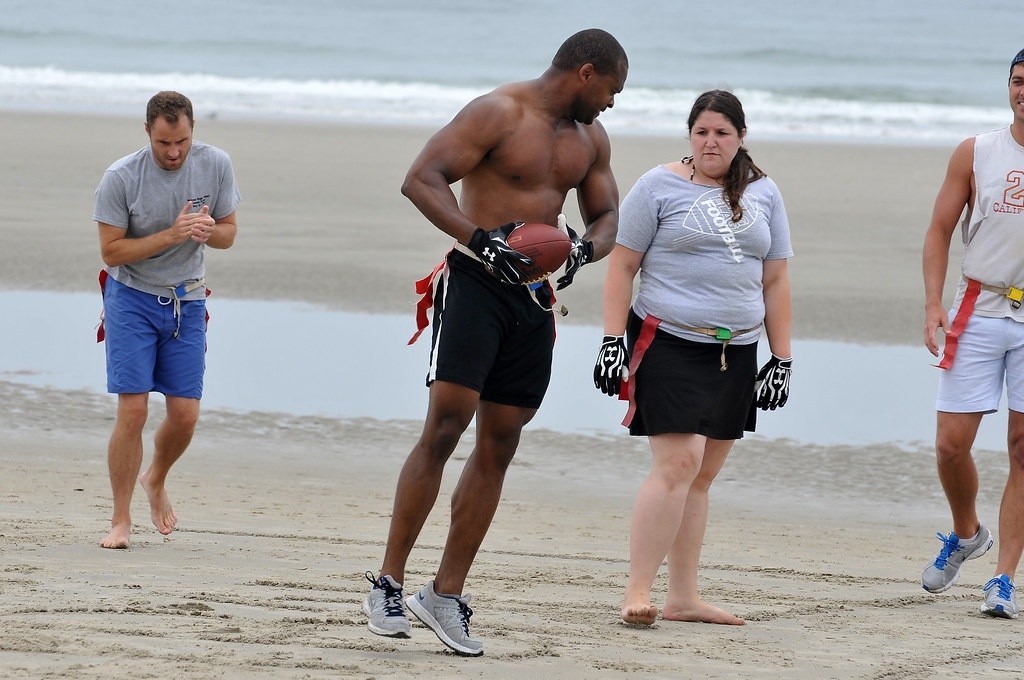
[99,90,242,546]
[922,49,1024,619]
[594,89,793,625]
[362,29,628,659]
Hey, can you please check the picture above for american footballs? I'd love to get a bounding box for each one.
[485,222,573,285]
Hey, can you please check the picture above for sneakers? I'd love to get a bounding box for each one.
[405,580,484,658]
[921,522,993,593]
[980,574,1019,619]
[362,571,411,638]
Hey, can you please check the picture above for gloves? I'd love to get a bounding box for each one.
[593,334,629,396]
[556,214,594,292]
[754,354,793,411]
[467,222,535,284]
